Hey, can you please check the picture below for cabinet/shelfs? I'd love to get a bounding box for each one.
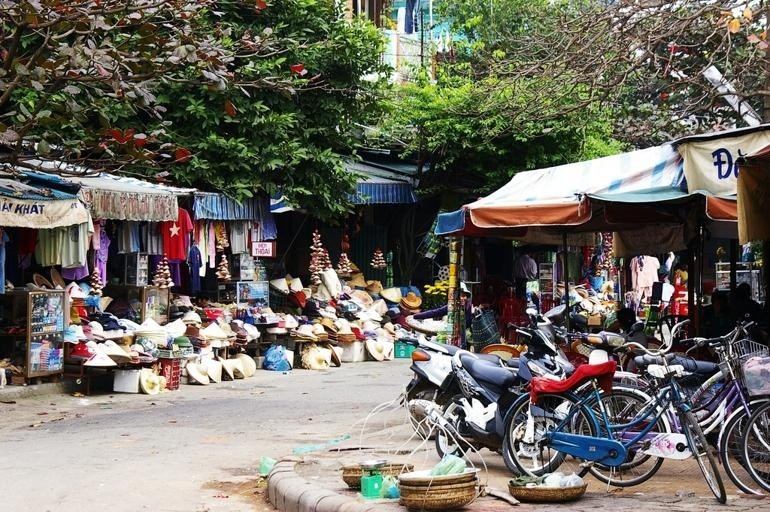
[0,290,65,384]
[217,280,271,307]
[103,285,170,326]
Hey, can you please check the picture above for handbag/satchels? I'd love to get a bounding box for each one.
[472,312,500,353]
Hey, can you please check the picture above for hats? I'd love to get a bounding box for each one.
[134,311,248,347]
[33,269,65,289]
[187,358,244,385]
[446,282,470,298]
[283,305,356,366]
[290,277,303,292]
[270,277,290,295]
[67,280,151,367]
[366,340,385,362]
[351,274,422,315]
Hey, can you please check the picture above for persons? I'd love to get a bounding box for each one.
[194,291,210,307]
[513,249,537,301]
[650,267,675,311]
[671,270,688,317]
[407,280,479,352]
[700,281,761,362]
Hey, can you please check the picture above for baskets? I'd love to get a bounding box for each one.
[342,465,587,508]
[720,339,770,397]
[644,357,703,409]
